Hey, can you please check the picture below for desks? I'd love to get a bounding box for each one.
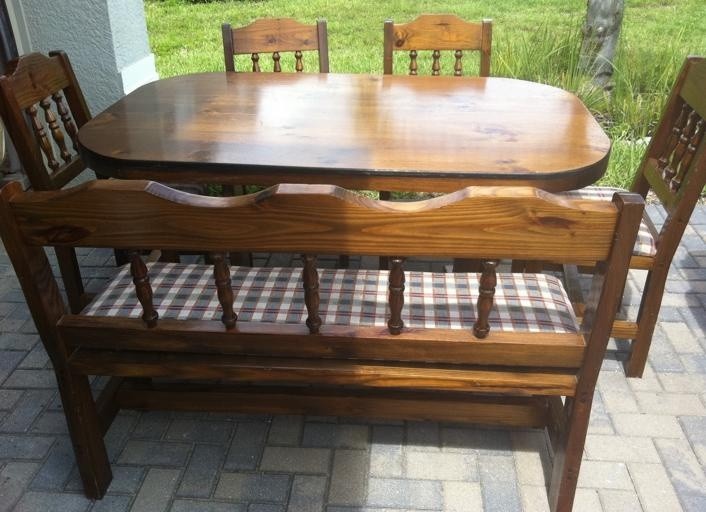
[76,72,610,268]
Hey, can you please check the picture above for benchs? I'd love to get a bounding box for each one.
[0,181,645,511]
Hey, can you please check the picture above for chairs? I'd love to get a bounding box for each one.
[0,50,205,315]
[512,55,706,378]
[221,17,350,262]
[379,13,493,273]
